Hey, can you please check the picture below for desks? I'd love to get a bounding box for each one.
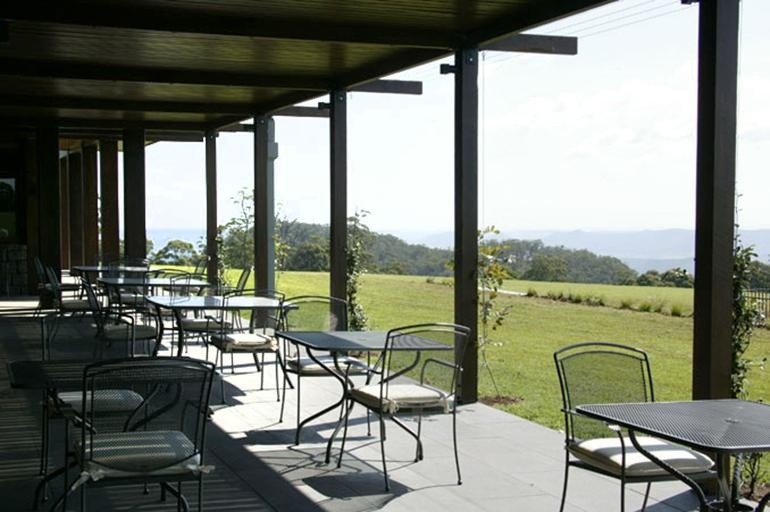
[37,362,220,505]
[275,328,453,463]
[577,398,770,512]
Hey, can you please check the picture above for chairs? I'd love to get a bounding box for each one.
[554,341,716,511]
[325,321,471,489]
[37,312,148,481]
[72,355,216,509]
[35,255,293,402]
[277,295,372,446]
